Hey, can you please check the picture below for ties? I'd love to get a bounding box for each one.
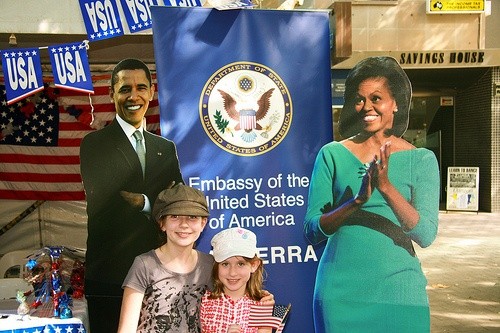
[133,130,146,181]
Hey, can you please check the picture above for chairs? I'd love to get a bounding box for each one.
[0,249,40,300]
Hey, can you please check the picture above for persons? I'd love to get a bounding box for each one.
[117,185,273,333]
[199,227,274,333]
[79,57,184,333]
[304,56,441,333]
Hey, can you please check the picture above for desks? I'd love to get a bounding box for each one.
[0,295,91,333]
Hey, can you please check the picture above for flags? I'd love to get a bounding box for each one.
[0,64,160,202]
[248,302,291,333]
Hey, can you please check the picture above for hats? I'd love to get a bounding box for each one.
[152,185,210,222]
[209,227,257,262]
